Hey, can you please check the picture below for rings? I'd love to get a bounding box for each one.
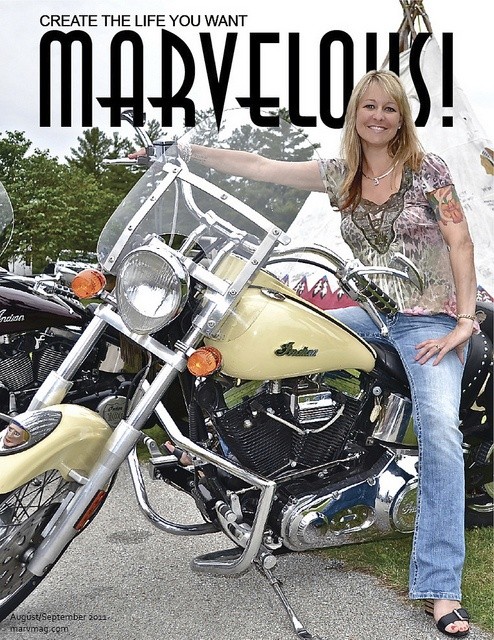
[435,345,441,351]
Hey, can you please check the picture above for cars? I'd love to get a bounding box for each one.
[47,251,98,275]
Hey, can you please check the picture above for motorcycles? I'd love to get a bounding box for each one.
[0,182,221,437]
[0,109,494,635]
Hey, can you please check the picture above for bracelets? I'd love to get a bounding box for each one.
[456,314,475,321]
[179,141,192,164]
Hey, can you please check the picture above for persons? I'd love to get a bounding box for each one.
[131,69,476,635]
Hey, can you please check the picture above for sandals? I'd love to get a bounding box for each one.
[425,592,470,639]
[161,442,191,468]
